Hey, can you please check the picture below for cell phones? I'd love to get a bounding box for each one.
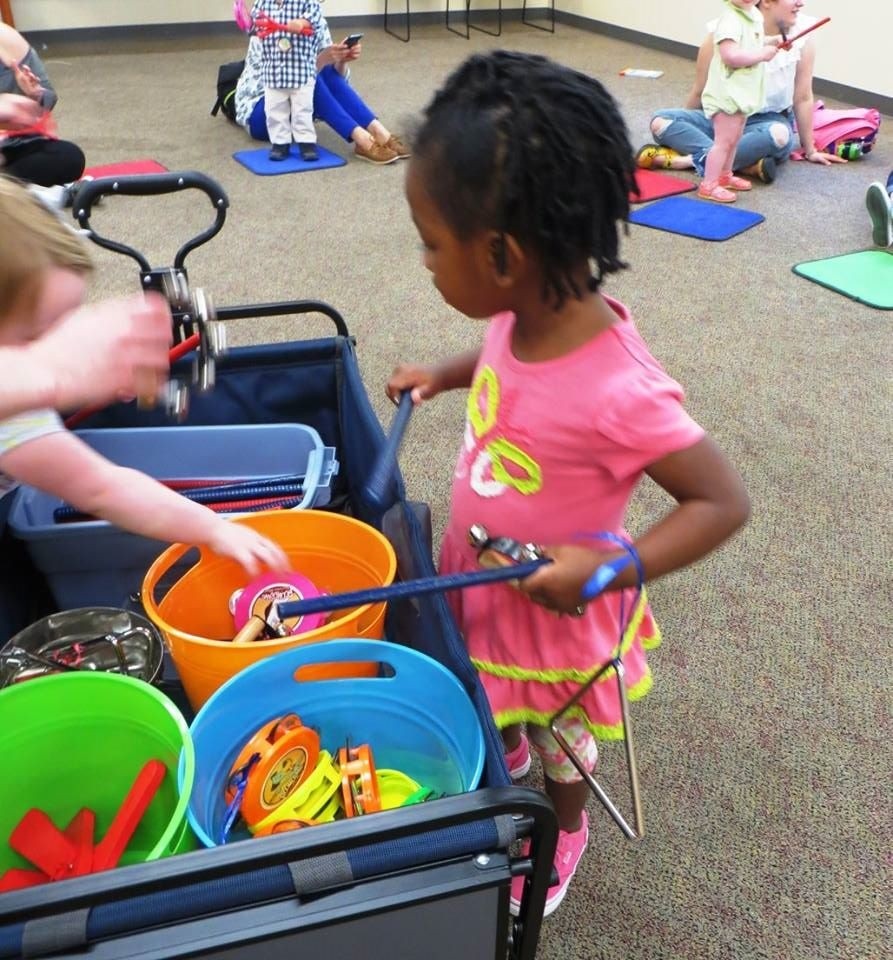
[340,32,363,49]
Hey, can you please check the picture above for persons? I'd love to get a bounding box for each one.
[0,173,294,579]
[634,0,849,203]
[0,22,85,188]
[866,171,893,247]
[389,49,755,918]
[234,0,413,166]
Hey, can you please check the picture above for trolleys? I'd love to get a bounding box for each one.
[0,168,562,960]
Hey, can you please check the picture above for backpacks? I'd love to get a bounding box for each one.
[792,99,879,161]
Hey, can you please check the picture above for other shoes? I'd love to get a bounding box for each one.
[64,174,103,207]
[270,144,289,160]
[300,143,317,160]
[637,144,679,168]
[758,158,776,183]
[866,182,892,247]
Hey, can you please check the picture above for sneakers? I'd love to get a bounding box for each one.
[355,142,399,164]
[504,731,532,778]
[386,135,413,158]
[509,811,588,920]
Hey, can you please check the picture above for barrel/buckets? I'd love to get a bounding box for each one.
[0,671,198,890]
[177,637,486,847]
[0,606,165,685]
[141,508,397,709]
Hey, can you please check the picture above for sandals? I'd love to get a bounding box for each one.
[696,180,737,202]
[719,172,751,190]
